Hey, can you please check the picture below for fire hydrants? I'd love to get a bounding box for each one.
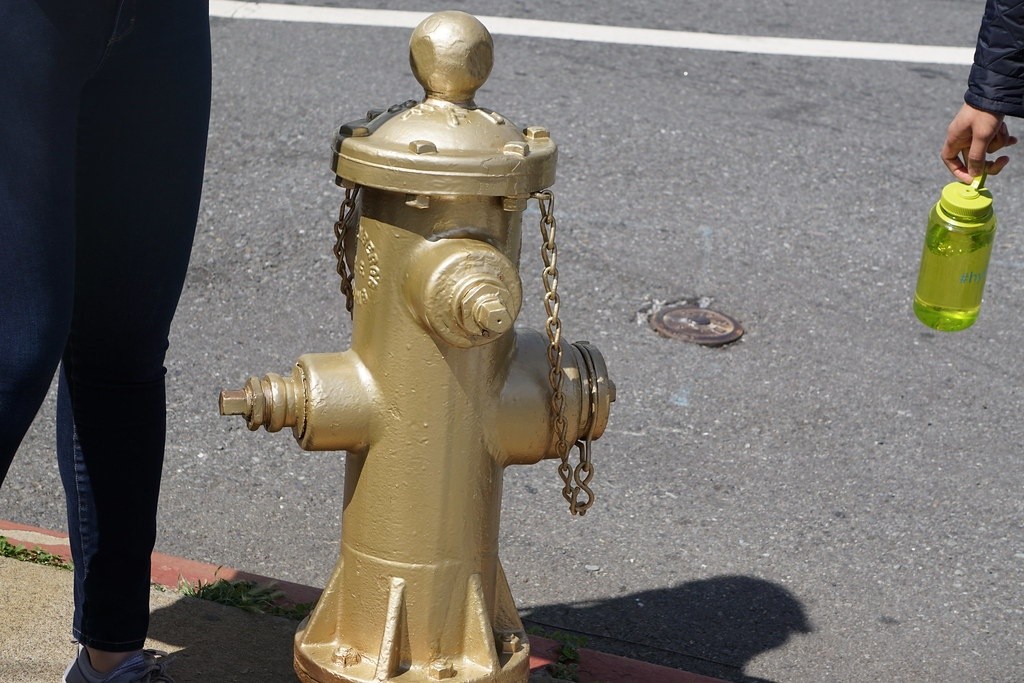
[220,11,615,683]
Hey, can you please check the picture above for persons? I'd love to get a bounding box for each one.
[939,0,1024,185]
[0,0,211,683]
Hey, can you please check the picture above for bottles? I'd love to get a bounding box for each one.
[912,161,997,331]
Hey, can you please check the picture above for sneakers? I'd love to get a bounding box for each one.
[63,649,176,683]
